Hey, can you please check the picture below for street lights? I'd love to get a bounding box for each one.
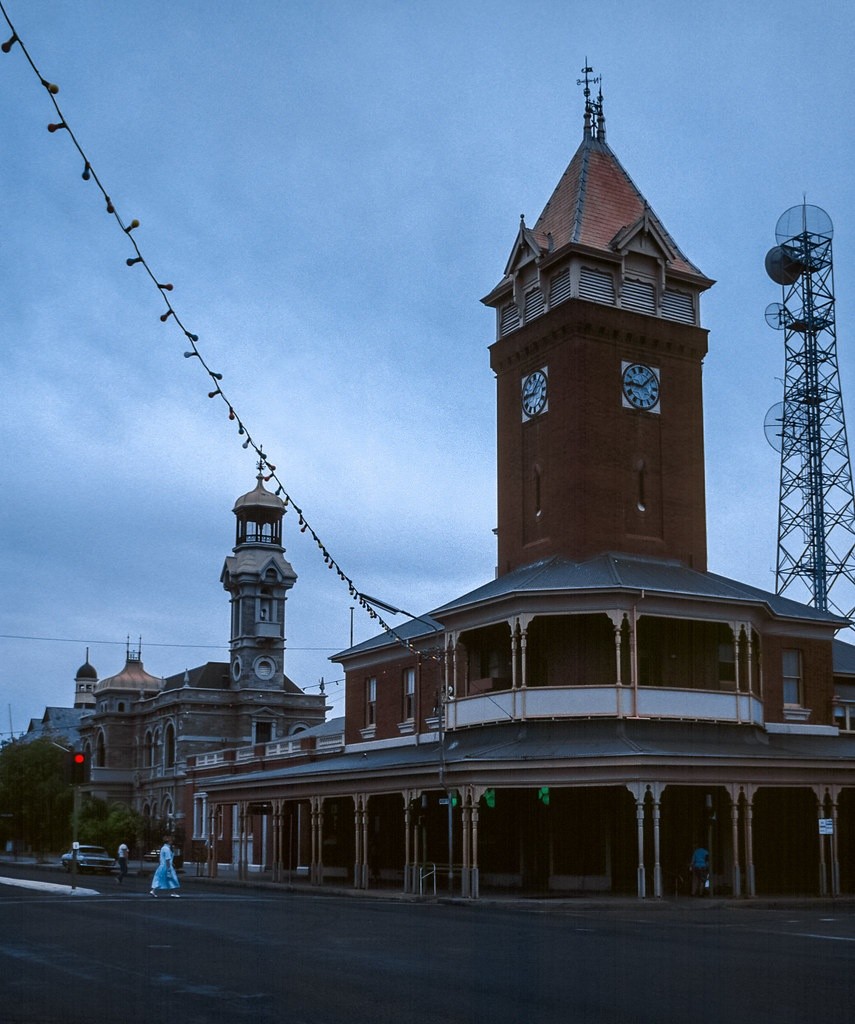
[354,590,455,898]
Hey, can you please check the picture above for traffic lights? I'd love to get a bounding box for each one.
[450,794,464,834]
[71,752,85,784]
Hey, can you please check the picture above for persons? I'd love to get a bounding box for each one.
[115,838,130,885]
[150,836,181,898]
[689,841,711,897]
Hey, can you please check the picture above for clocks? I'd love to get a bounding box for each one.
[622,362,660,409]
[520,370,548,417]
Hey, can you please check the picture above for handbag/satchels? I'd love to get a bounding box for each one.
[705,880,709,888]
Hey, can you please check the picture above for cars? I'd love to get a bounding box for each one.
[59,844,115,874]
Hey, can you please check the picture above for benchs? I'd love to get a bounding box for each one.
[144,849,161,862]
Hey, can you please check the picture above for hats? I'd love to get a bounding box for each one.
[162,835,172,843]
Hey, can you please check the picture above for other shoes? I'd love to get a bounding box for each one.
[113,877,120,884]
[170,894,180,897]
[149,891,158,897]
